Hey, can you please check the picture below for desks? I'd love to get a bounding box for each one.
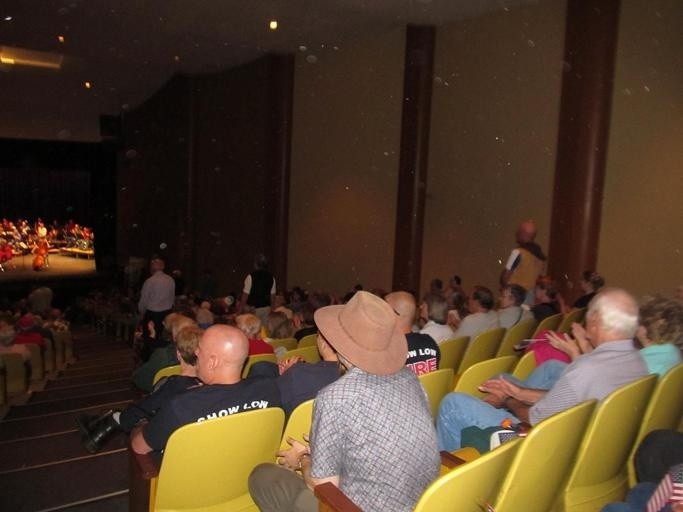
[64,248,94,260]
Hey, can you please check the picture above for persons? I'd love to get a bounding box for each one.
[0,215,94,395]
[497,221,549,306]
[86,259,682,511]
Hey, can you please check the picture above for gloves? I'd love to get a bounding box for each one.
[243,275,252,294]
[271,279,276,295]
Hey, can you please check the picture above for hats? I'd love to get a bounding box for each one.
[314,291,408,375]
[17,313,36,328]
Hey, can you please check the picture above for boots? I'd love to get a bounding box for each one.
[73,408,122,453]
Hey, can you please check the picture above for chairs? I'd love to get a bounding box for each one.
[0,331,71,418]
